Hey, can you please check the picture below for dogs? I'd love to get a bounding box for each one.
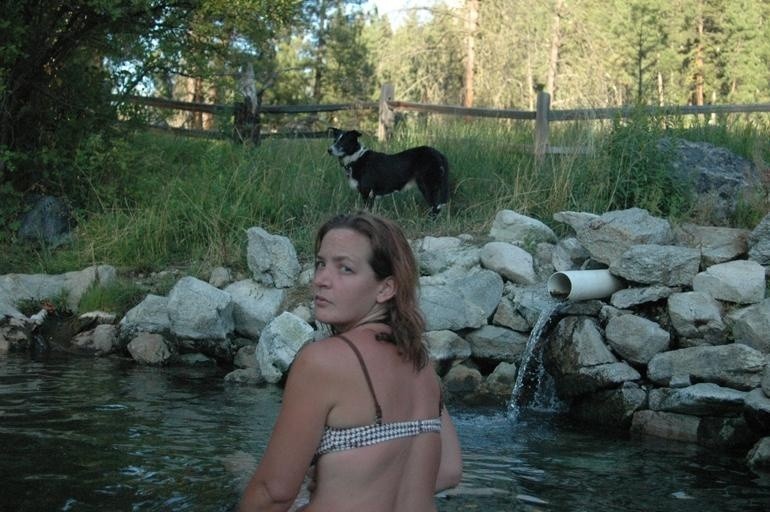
[326,127,451,223]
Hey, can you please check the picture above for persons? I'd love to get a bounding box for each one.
[240,210,462,511]
